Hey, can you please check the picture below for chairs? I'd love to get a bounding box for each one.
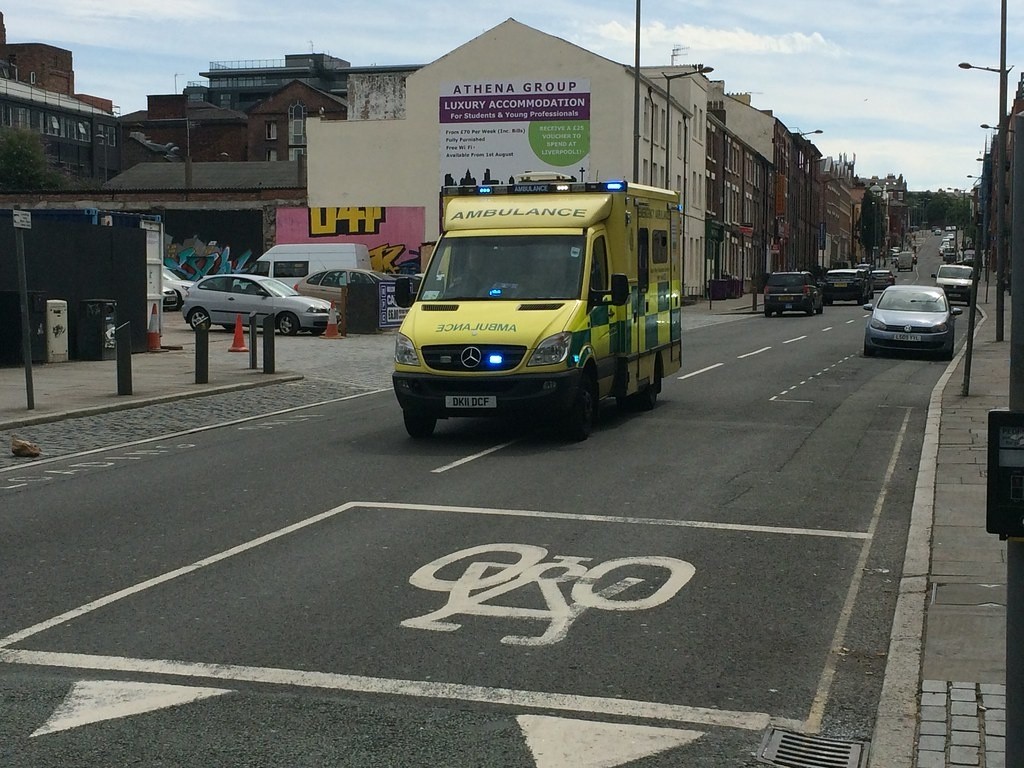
[234,284,241,293]
[247,285,258,294]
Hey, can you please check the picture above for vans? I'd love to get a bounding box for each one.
[898,252,914,272]
[246,243,372,289]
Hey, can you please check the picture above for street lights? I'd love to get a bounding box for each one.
[787,129,823,270]
[665,66,715,190]
[851,202,876,267]
[958,63,1008,345]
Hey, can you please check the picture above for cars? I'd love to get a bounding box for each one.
[931,265,974,304]
[162,264,198,310]
[161,286,178,309]
[294,268,396,326]
[931,226,958,264]
[871,270,898,290]
[388,272,445,299]
[891,253,899,264]
[863,284,964,363]
[911,254,918,264]
[856,265,872,273]
[183,274,331,336]
[891,249,899,253]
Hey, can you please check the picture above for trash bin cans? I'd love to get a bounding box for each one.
[79,298,117,361]
[708,279,727,300]
[29,300,69,363]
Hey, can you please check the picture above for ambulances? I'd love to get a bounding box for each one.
[391,179,685,441]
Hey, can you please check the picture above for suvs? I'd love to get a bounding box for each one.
[823,268,869,304]
[763,270,824,317]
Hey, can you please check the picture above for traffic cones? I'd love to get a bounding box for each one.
[227,315,249,351]
[318,301,345,339]
[148,303,169,352]
